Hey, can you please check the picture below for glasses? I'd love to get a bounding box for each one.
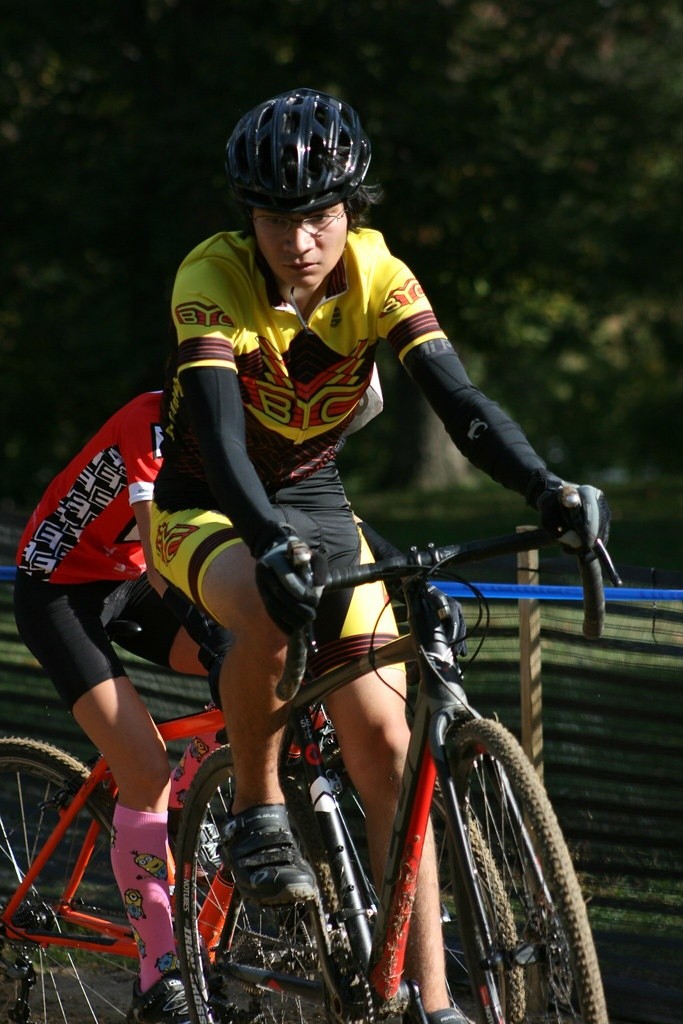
[243,210,335,233]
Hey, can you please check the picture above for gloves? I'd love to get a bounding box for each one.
[522,467,612,564]
[255,517,328,636]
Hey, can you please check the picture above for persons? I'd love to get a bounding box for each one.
[13,378,466,1024]
[151,89,611,1024]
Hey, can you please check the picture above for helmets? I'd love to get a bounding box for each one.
[222,88,373,215]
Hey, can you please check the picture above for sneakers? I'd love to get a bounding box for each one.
[215,799,318,906]
[401,995,469,1024]
[125,968,216,1024]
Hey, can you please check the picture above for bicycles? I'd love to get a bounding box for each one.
[0,699,529,1024]
[175,486,622,1024]
